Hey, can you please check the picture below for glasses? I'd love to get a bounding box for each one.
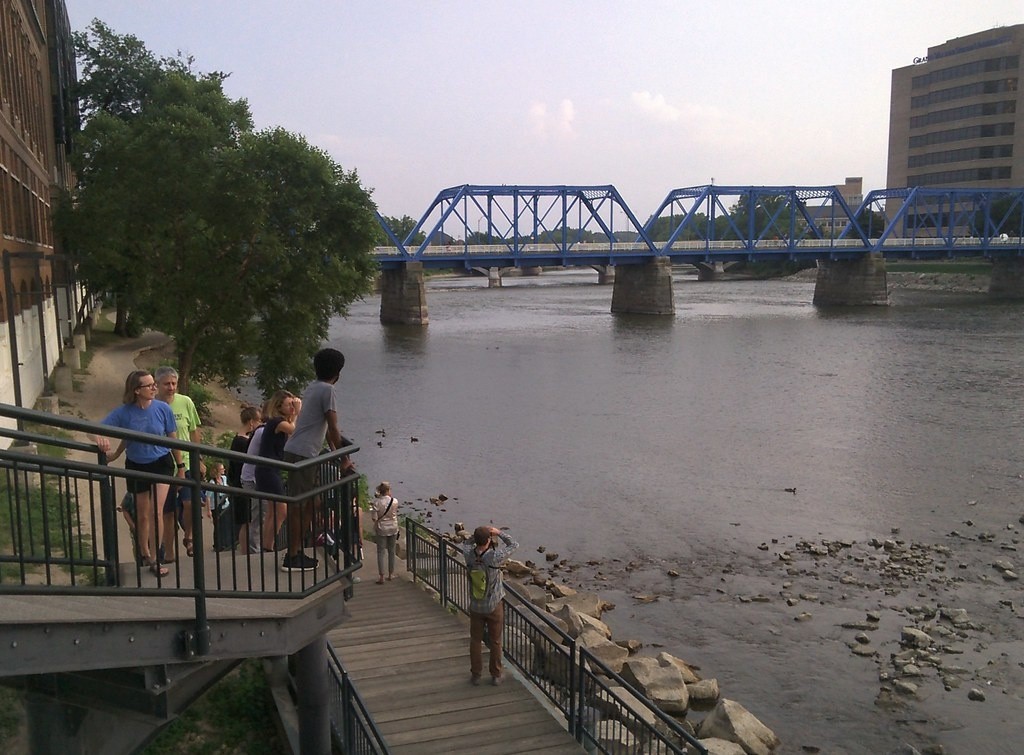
[141,383,157,389]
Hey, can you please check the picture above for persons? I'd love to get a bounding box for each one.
[463,527,519,686]
[372,482,400,584]
[281,347,354,570]
[255,389,301,553]
[122,491,152,566]
[229,407,261,553]
[86,370,187,576]
[206,462,230,552]
[240,398,269,555]
[153,366,206,564]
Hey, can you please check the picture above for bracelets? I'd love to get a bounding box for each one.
[199,454,203,460]
[177,463,185,469]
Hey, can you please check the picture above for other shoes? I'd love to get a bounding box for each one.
[281,554,318,571]
[493,674,505,685]
[284,552,320,564]
[472,677,480,685]
[353,577,360,583]
[376,580,384,584]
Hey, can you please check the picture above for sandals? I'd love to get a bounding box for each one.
[183,538,194,557]
[143,555,154,566]
[150,563,169,577]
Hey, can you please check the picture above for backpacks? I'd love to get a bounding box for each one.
[468,547,489,600]
